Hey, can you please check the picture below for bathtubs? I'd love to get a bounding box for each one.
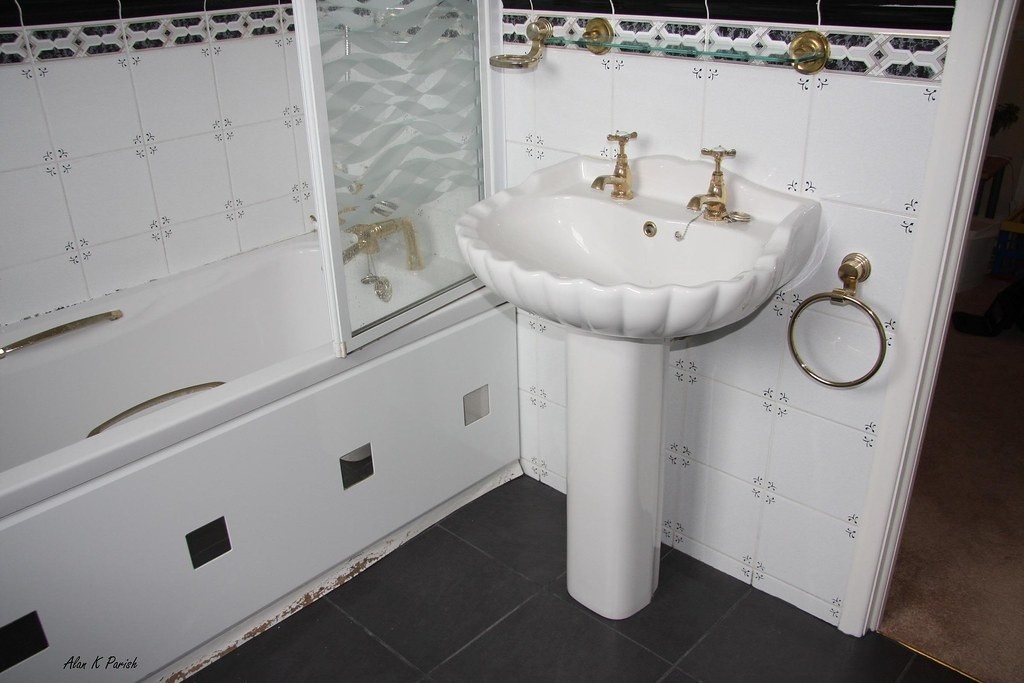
[1,220,520,682]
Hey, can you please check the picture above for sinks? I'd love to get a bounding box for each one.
[456,152,822,340]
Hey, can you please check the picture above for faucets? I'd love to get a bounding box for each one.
[688,146,743,223]
[592,131,642,198]
[339,215,423,272]
